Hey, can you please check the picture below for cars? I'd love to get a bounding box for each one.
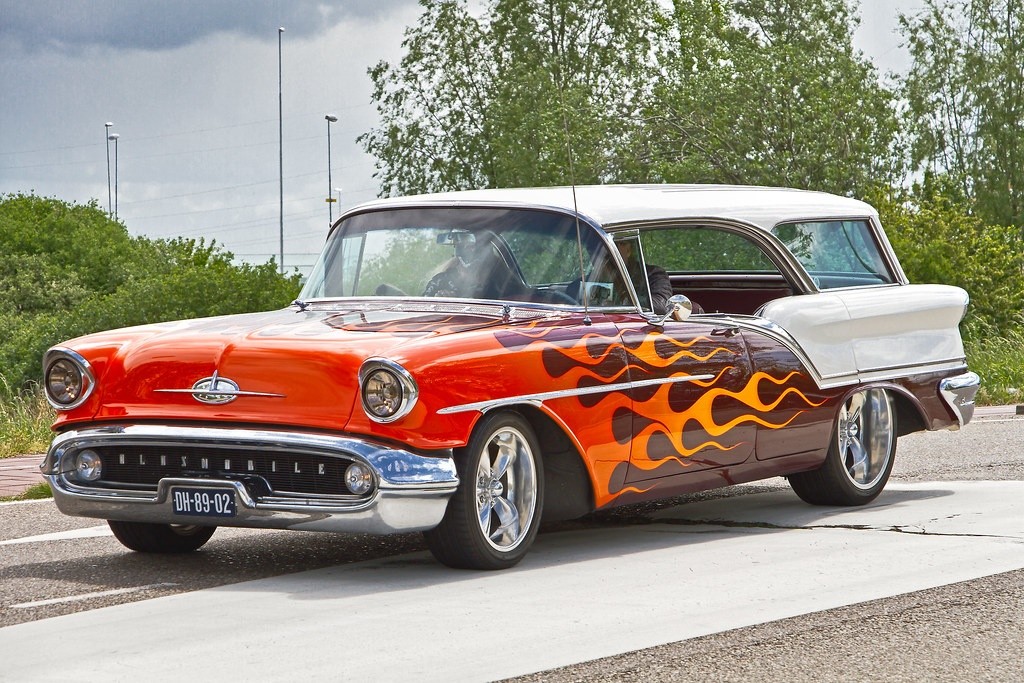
[37,181,985,573]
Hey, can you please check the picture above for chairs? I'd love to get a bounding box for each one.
[423,242,526,302]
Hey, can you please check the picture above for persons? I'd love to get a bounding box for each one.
[422,235,521,301]
[564,229,674,317]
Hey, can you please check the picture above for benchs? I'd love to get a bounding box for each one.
[565,275,820,319]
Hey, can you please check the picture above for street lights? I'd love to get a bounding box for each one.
[323,115,337,227]
[333,187,342,219]
[276,27,287,277]
[105,122,115,219]
[108,133,121,220]
